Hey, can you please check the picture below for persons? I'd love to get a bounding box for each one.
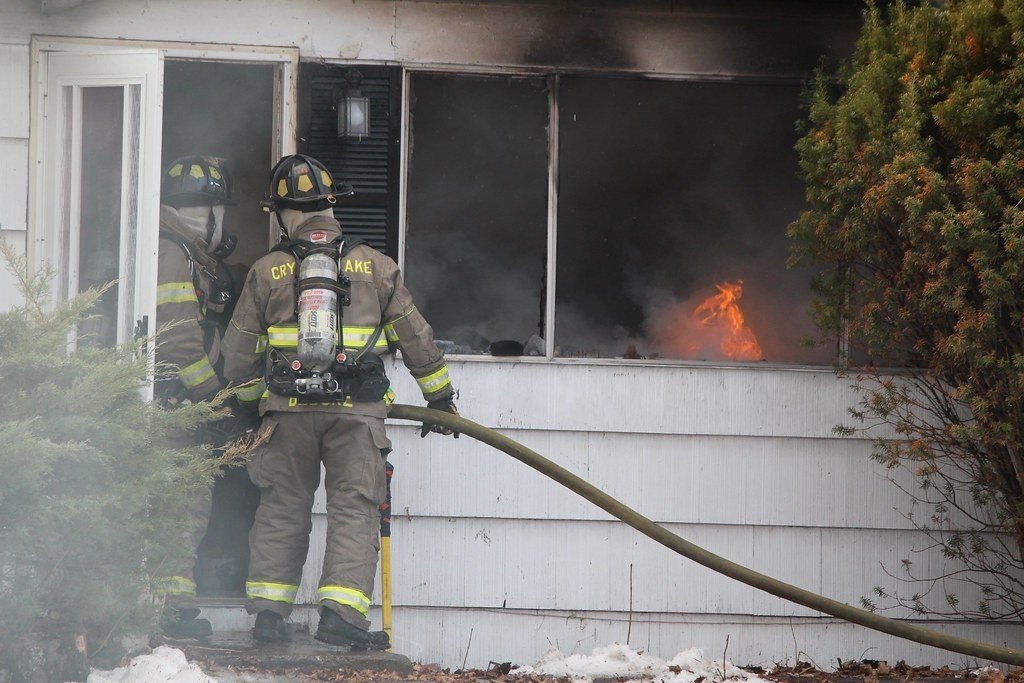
[218,155,460,650]
[84,153,253,639]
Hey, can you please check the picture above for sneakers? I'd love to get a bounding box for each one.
[314,607,391,649]
[159,608,213,639]
[253,610,293,641]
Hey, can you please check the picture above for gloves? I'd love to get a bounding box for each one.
[232,398,262,435]
[193,387,232,436]
[420,398,459,439]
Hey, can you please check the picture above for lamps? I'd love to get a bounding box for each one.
[325,67,371,141]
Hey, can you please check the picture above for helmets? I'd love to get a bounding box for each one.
[264,154,356,213]
[161,156,237,208]
